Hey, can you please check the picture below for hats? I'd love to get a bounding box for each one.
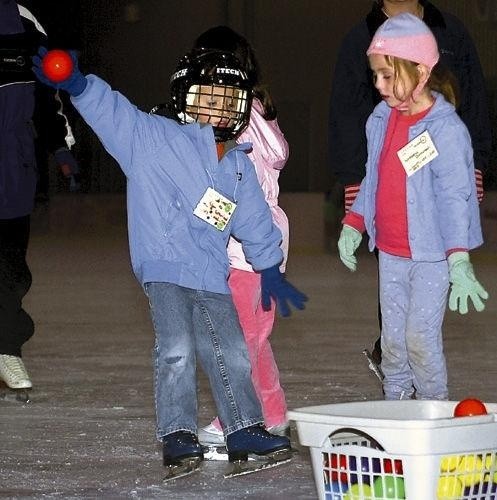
[366,12,439,69]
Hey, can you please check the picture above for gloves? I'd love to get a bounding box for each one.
[337,225,362,272]
[55,147,82,191]
[475,169,483,202]
[261,264,309,317]
[448,252,489,314]
[32,47,87,97]
[345,183,360,214]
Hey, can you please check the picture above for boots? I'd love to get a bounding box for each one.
[197,422,290,446]
[162,430,203,467]
[226,424,290,462]
[0,354,32,389]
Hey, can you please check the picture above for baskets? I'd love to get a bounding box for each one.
[287,400,497,500]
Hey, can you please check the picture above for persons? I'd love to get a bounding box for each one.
[31,44,294,481]
[194,24,291,462]
[0,0,80,402]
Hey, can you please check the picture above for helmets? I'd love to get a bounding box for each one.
[170,47,254,113]
[193,26,251,48]
